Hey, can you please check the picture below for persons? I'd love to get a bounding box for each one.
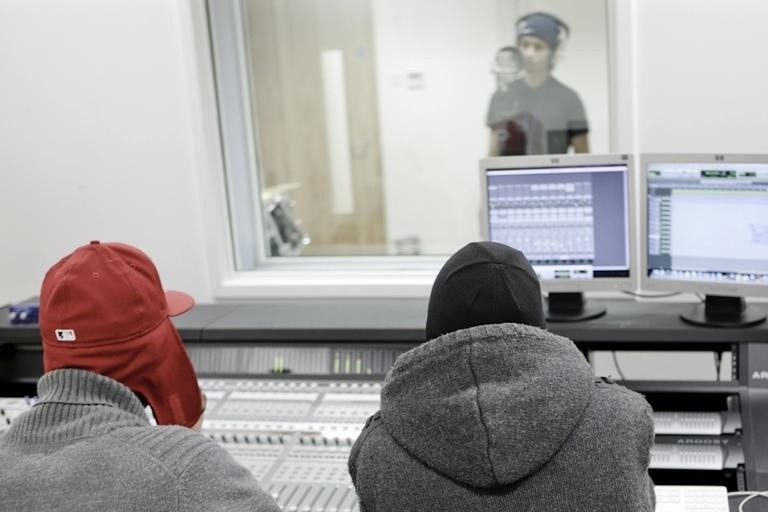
[346,241,657,512]
[486,12,588,155]
[0,238,283,512]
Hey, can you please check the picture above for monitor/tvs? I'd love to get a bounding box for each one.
[477,151,638,325]
[638,151,768,329]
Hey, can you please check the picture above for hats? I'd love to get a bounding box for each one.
[38,242,202,427]
[426,242,546,342]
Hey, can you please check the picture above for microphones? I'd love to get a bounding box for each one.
[493,43,523,84]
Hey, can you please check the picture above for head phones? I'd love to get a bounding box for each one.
[513,8,573,73]
